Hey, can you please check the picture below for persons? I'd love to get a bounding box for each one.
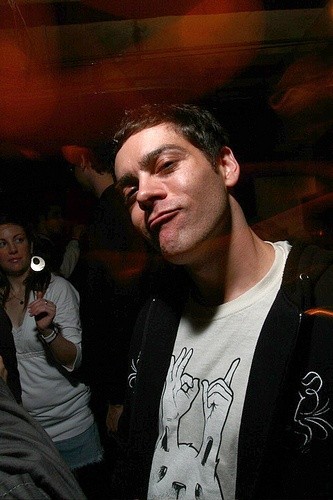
[105,263,182,449]
[0,216,103,499]
[0,354,87,500]
[0,129,332,288]
[105,100,332,500]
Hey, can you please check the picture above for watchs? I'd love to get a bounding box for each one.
[36,325,59,345]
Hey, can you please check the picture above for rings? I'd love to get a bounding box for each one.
[43,299,47,304]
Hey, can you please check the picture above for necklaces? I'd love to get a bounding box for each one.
[9,290,24,305]
